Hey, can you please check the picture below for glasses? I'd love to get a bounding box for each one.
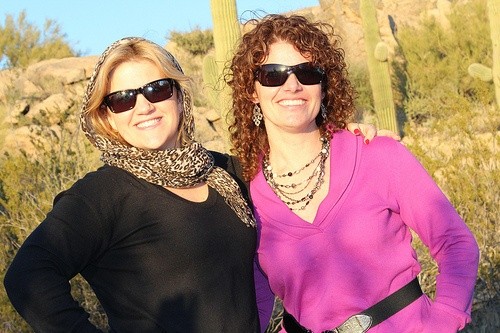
[102,78,179,114]
[256,62,324,87]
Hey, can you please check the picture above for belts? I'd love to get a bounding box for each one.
[283,277,423,333]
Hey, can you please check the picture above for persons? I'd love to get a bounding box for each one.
[2,36,410,333]
[217,12,482,333]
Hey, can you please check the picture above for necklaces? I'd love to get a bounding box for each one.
[259,127,332,211]
